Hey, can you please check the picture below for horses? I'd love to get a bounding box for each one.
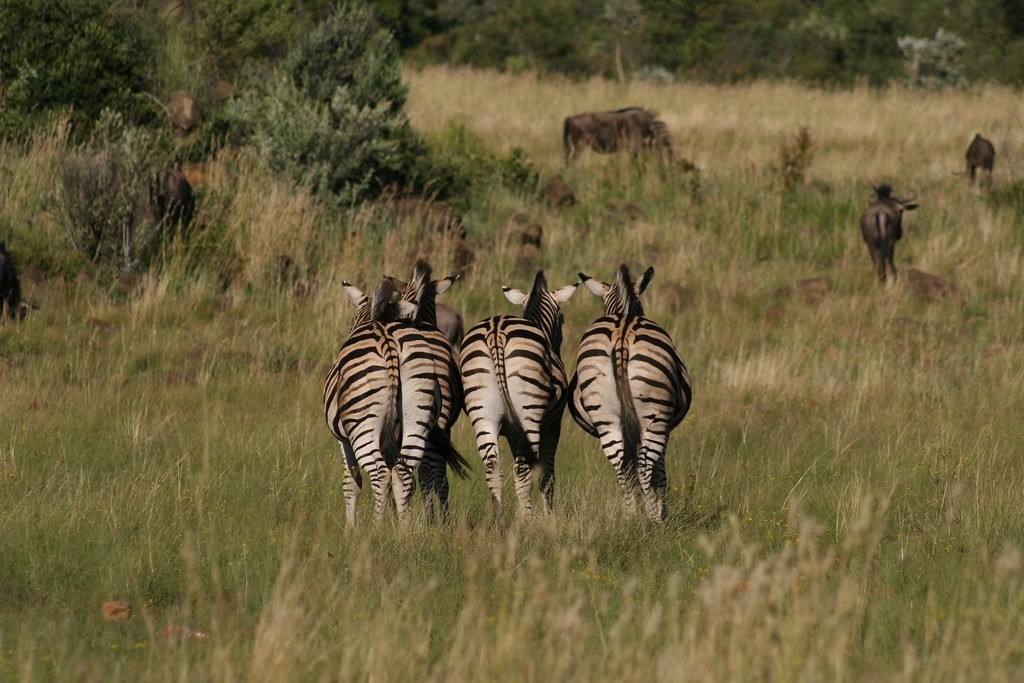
[562,107,676,167]
[435,302,464,355]
[966,132,996,185]
[148,168,194,251]
[860,185,921,291]
[0,239,29,324]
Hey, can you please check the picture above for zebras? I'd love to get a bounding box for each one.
[322,259,472,534]
[459,270,581,521]
[566,264,692,523]
